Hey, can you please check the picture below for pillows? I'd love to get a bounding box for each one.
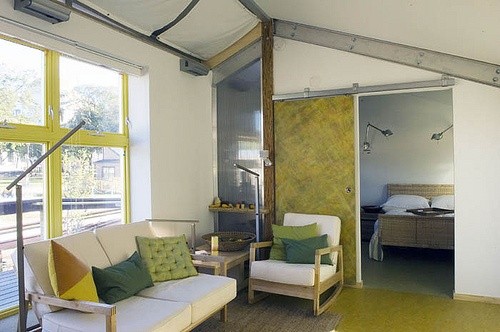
[430,195,454,210]
[91,250,154,305]
[47,240,99,314]
[268,222,318,260]
[136,234,198,282]
[280,234,334,265]
[380,194,429,210]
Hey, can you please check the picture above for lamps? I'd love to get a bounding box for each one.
[234,163,262,261]
[362,123,394,153]
[259,150,272,167]
[430,125,453,141]
[6,121,86,331]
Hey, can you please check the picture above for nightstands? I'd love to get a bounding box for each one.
[361,211,383,241]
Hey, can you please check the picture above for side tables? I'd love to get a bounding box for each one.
[192,243,251,291]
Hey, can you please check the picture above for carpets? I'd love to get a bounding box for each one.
[323,284,499,331]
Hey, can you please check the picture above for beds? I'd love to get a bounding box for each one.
[377,183,454,263]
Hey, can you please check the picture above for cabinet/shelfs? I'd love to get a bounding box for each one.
[208,204,268,215]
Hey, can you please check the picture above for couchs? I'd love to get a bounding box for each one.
[17,220,238,332]
[248,212,344,317]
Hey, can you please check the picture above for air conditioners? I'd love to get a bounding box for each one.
[14,0,71,25]
[180,59,210,77]
